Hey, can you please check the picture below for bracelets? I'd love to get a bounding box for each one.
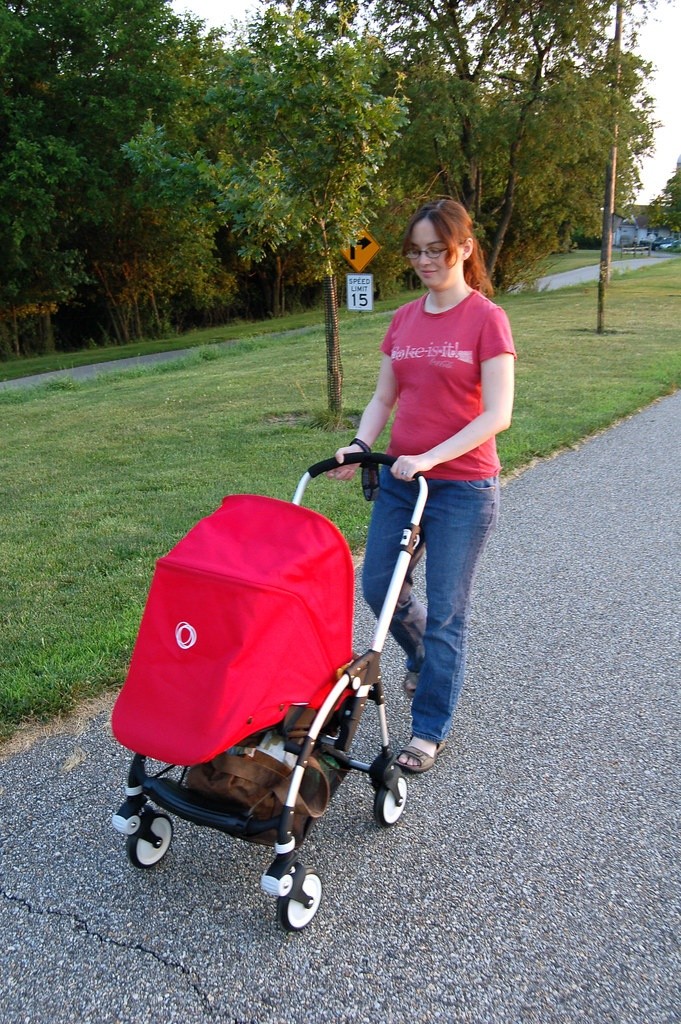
[350,437,371,453]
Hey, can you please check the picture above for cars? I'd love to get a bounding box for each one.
[639,233,681,250]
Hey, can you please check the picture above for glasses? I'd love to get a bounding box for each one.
[404,242,460,259]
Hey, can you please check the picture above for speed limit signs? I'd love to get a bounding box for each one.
[345,273,374,313]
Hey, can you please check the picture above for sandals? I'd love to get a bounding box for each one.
[404,671,419,697]
[394,735,446,773]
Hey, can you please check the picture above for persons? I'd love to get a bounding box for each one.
[324,197,517,771]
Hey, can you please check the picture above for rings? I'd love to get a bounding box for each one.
[400,471,406,476]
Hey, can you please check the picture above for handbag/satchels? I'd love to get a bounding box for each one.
[184,734,330,819]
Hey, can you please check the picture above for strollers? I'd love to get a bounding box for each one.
[112,451,409,932]
